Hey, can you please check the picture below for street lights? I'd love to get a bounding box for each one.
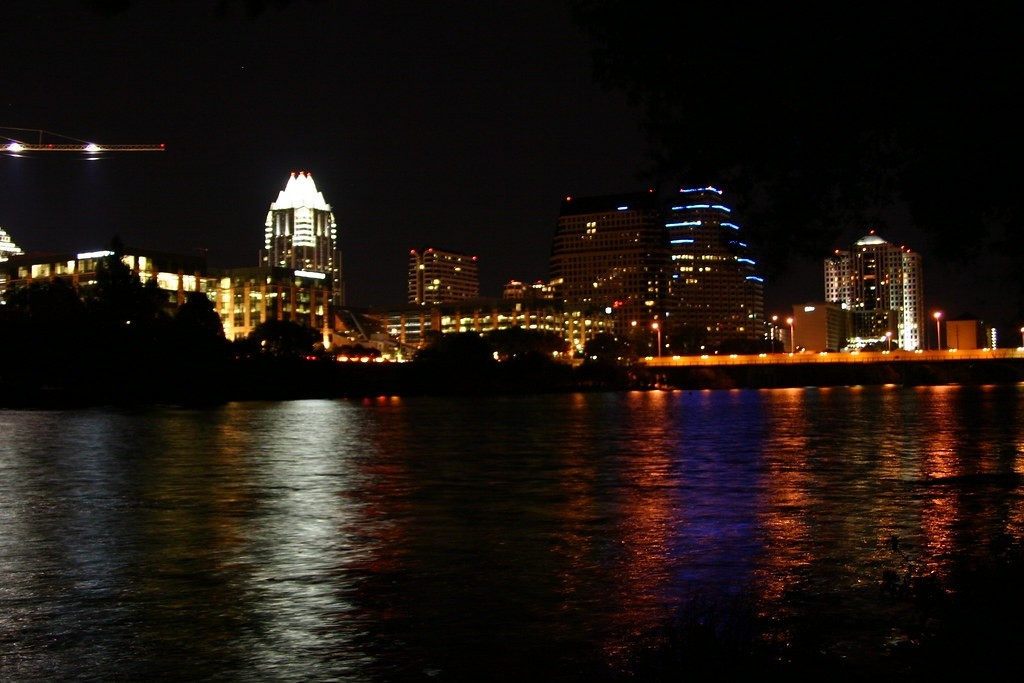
[886,332,891,352]
[933,311,941,351]
[787,318,794,352]
[1020,327,1024,347]
[651,322,661,356]
